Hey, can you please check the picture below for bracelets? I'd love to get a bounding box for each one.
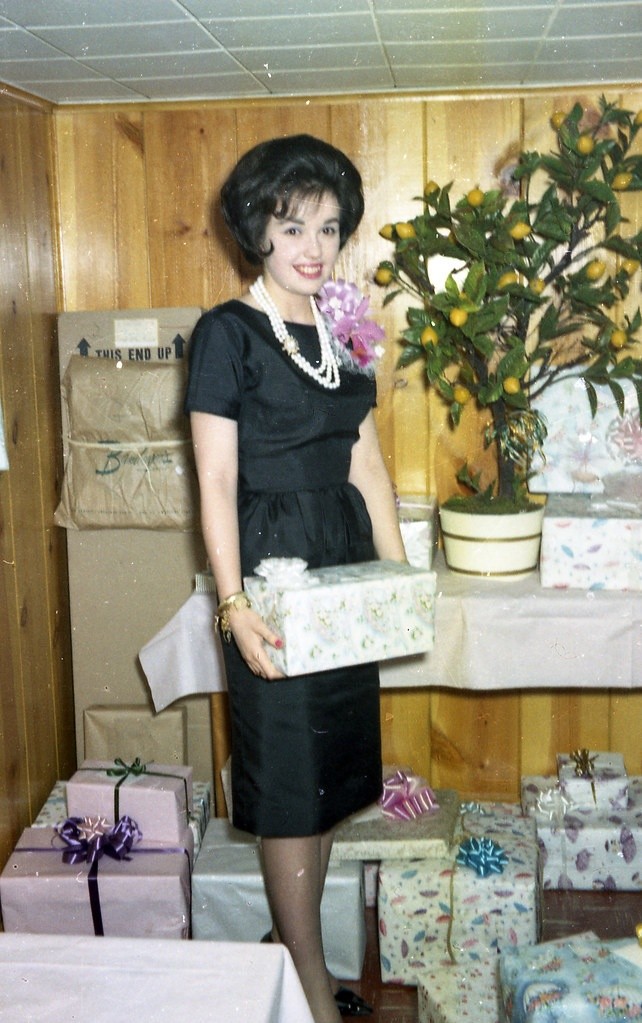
[214,590,254,641]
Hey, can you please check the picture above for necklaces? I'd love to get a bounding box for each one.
[248,274,341,390]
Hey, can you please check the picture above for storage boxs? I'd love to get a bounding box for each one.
[538,495,642,592]
[58,306,215,818]
[83,704,189,766]
[525,365,642,496]
[65,758,194,843]
[397,493,440,570]
[0,827,196,940]
[32,779,211,864]
[243,558,438,677]
[189,751,642,1023]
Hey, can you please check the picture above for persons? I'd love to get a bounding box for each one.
[184,136,414,1023]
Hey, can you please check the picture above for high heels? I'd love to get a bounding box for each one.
[259,931,374,1018]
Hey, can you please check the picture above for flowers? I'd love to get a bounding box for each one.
[254,556,310,585]
[316,279,385,367]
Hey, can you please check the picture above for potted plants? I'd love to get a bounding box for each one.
[373,92,642,583]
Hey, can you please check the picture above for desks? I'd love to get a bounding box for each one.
[0,932,315,1023]
[138,549,642,803]
